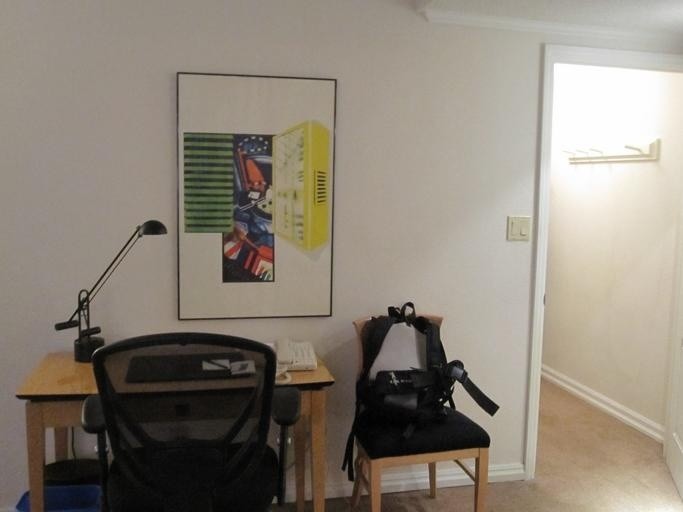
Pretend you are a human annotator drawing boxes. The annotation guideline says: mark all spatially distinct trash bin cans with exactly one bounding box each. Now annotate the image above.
[15,458,108,512]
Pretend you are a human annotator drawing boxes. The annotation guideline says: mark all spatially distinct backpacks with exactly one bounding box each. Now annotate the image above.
[354,302,447,435]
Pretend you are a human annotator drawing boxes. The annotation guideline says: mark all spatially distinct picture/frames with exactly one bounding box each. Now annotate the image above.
[177,73,338,321]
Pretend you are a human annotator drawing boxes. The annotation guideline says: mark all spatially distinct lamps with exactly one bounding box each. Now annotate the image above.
[54,213,170,364]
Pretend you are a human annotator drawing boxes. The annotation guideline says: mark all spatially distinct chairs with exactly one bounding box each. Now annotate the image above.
[80,331,300,511]
[349,309,492,510]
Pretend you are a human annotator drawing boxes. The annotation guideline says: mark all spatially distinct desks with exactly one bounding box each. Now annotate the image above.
[16,351,337,512]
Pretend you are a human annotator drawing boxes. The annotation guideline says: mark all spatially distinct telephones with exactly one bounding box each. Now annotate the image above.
[264,339,318,371]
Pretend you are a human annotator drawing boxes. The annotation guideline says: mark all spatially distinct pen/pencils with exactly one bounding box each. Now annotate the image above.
[203,359,228,370]
